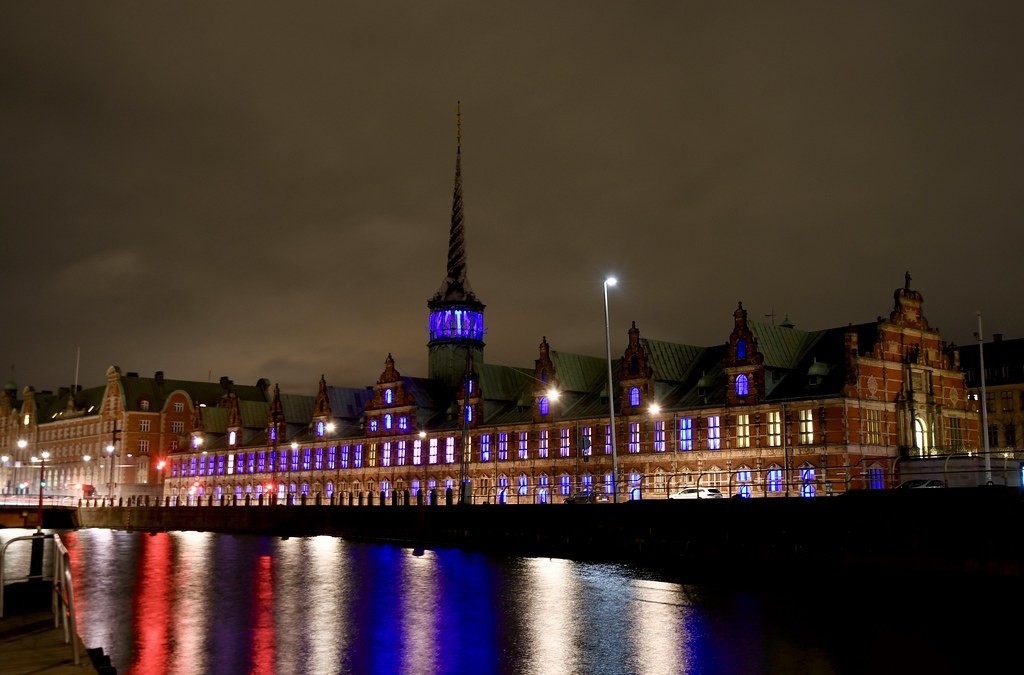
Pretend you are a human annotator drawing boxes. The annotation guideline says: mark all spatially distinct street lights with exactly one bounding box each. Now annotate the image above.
[603,276,618,502]
[976,310,993,486]
